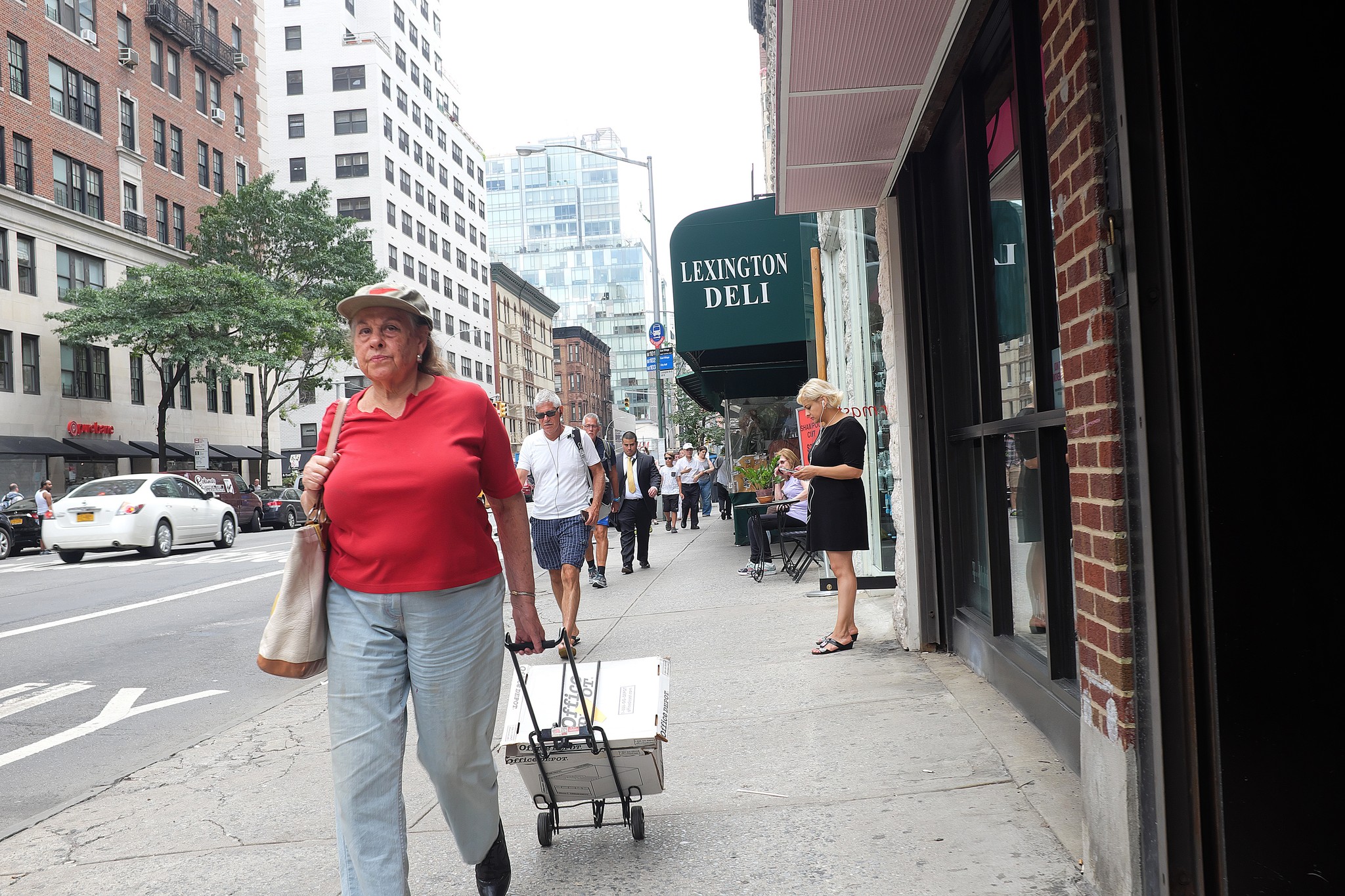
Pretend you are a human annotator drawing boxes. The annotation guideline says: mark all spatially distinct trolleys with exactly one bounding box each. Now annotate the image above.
[501,628,646,846]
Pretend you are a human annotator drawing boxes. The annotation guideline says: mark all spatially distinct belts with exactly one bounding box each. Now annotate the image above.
[682,483,698,487]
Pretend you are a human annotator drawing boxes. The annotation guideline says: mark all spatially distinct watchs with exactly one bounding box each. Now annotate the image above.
[613,497,621,502]
[697,474,701,477]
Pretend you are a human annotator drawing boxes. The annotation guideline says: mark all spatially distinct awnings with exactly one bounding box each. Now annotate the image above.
[675,195,820,417]
[988,196,1034,347]
[0,437,289,460]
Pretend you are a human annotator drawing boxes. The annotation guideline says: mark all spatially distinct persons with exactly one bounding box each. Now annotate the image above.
[299,280,539,896]
[579,412,621,588]
[615,431,662,574]
[640,446,661,525]
[34,479,54,555]
[675,443,704,529]
[1013,379,1047,635]
[713,446,733,520]
[736,447,810,577]
[695,446,715,517]
[514,388,606,659]
[789,377,868,655]
[252,479,262,493]
[1,483,25,510]
[659,452,685,533]
[678,448,686,458]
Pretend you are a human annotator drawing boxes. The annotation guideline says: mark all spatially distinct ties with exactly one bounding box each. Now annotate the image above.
[627,456,636,494]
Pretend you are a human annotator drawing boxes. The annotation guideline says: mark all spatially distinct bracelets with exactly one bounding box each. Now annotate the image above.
[509,590,536,598]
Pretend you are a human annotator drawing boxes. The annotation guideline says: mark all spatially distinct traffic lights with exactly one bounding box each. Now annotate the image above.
[499,402,507,417]
[624,397,630,411]
[492,403,497,413]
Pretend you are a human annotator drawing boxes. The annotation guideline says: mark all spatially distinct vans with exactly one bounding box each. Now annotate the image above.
[157,470,264,533]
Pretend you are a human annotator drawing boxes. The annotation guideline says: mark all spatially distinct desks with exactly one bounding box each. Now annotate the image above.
[734,499,800,583]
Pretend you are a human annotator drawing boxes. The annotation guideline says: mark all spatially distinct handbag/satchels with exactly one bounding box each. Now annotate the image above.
[607,452,642,528]
[257,397,351,678]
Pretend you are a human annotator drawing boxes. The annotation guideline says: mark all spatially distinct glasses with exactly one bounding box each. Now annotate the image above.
[640,449,647,452]
[664,457,673,460]
[535,406,560,419]
[777,459,788,464]
[582,424,598,428]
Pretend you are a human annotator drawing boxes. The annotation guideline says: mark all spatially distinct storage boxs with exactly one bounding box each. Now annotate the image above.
[732,454,767,475]
[733,472,767,492]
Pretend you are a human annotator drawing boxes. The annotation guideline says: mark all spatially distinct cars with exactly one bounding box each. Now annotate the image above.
[0,493,70,557]
[42,474,238,564]
[0,512,16,560]
[253,488,317,530]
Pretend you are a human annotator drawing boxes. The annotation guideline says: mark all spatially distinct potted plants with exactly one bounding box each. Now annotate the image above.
[733,455,787,505]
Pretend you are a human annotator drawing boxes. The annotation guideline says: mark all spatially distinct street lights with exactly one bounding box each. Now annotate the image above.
[605,416,628,441]
[515,143,667,438]
[439,329,481,360]
[635,423,657,434]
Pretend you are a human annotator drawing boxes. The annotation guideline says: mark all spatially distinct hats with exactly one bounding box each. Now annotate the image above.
[683,443,694,450]
[336,280,433,331]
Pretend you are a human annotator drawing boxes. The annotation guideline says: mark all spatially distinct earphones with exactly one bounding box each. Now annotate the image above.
[822,400,825,407]
[560,412,561,416]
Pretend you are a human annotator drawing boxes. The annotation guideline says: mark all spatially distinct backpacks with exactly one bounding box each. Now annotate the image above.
[2,494,24,511]
[585,481,613,522]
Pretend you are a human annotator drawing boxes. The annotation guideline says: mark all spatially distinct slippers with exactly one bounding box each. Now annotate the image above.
[571,636,580,646]
[812,638,854,655]
[816,631,859,645]
[558,642,576,658]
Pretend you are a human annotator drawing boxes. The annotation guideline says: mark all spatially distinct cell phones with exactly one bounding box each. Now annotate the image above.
[580,510,589,521]
[780,468,797,474]
[778,469,784,475]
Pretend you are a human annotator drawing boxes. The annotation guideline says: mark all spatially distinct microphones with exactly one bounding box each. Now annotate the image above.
[559,436,560,440]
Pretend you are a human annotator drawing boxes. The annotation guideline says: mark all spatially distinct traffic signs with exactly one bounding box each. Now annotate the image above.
[646,347,674,372]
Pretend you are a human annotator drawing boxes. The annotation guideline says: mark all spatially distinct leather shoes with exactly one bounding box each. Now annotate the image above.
[475,815,512,896]
[654,523,658,525]
[622,565,633,574]
[681,521,687,528]
[727,516,732,519]
[721,510,726,520]
[691,526,700,529]
[639,561,650,568]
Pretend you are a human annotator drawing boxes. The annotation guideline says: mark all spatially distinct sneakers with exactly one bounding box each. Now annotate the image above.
[592,574,607,588]
[634,530,637,537]
[737,559,761,576]
[666,517,672,531]
[747,560,777,577]
[588,564,597,584]
[39,549,57,555]
[671,527,677,533]
[649,526,652,532]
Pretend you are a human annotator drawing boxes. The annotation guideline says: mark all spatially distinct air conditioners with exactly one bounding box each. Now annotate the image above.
[234,53,249,67]
[235,125,244,136]
[82,29,97,44]
[211,108,225,122]
[118,48,139,65]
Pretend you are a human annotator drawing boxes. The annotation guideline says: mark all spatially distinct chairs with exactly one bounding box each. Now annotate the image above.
[780,527,828,565]
[780,530,824,584]
